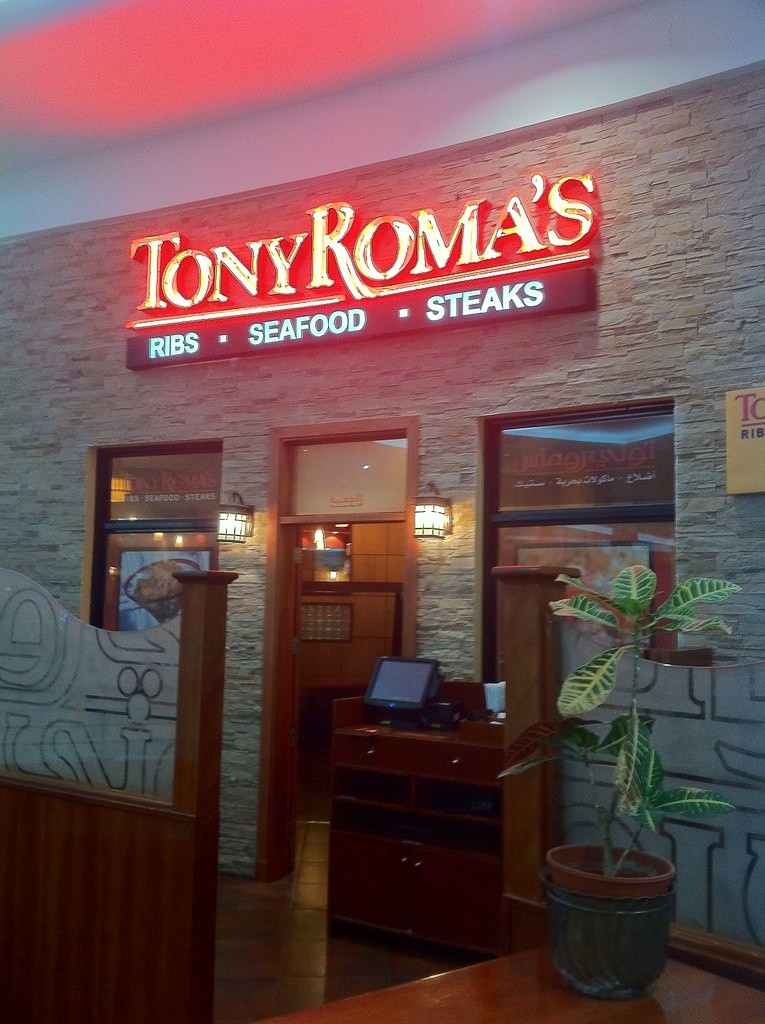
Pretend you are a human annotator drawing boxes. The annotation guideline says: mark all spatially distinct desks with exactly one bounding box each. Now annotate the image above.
[251,943,765,1024]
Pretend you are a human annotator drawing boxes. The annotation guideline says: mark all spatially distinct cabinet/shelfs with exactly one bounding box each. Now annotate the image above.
[325,683,502,964]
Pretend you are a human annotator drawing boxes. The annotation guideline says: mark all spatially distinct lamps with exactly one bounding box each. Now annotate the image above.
[216,493,253,544]
[414,481,453,538]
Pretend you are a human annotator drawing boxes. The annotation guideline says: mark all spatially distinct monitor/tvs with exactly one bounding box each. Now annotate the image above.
[364,655,437,709]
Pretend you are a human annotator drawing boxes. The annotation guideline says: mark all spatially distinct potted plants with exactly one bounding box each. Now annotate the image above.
[495,565,743,1000]
